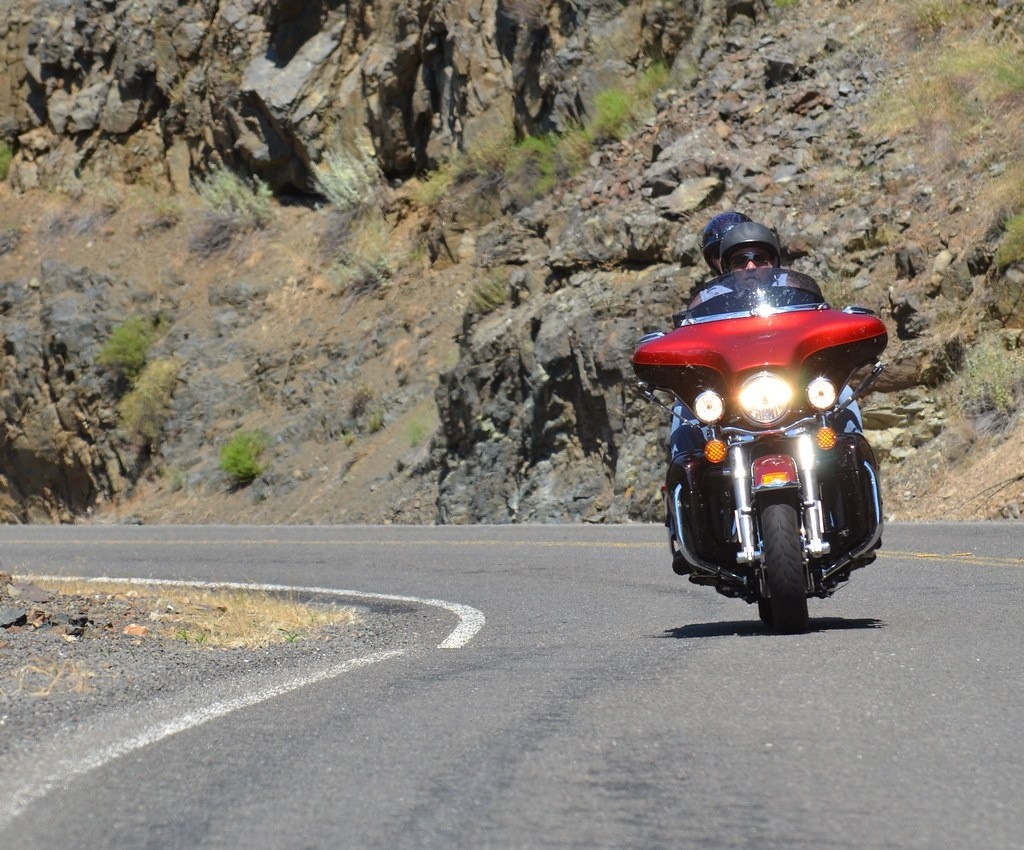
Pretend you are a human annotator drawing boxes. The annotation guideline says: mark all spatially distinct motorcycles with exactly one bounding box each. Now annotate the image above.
[628,267,889,635]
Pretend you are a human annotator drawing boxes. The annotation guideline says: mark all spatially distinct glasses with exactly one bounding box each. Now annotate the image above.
[732,251,771,266]
[712,249,720,259]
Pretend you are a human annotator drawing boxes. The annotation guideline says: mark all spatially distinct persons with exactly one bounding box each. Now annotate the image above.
[668,212,878,576]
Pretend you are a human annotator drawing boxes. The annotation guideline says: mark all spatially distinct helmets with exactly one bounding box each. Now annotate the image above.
[702,212,752,268]
[721,221,782,272]
